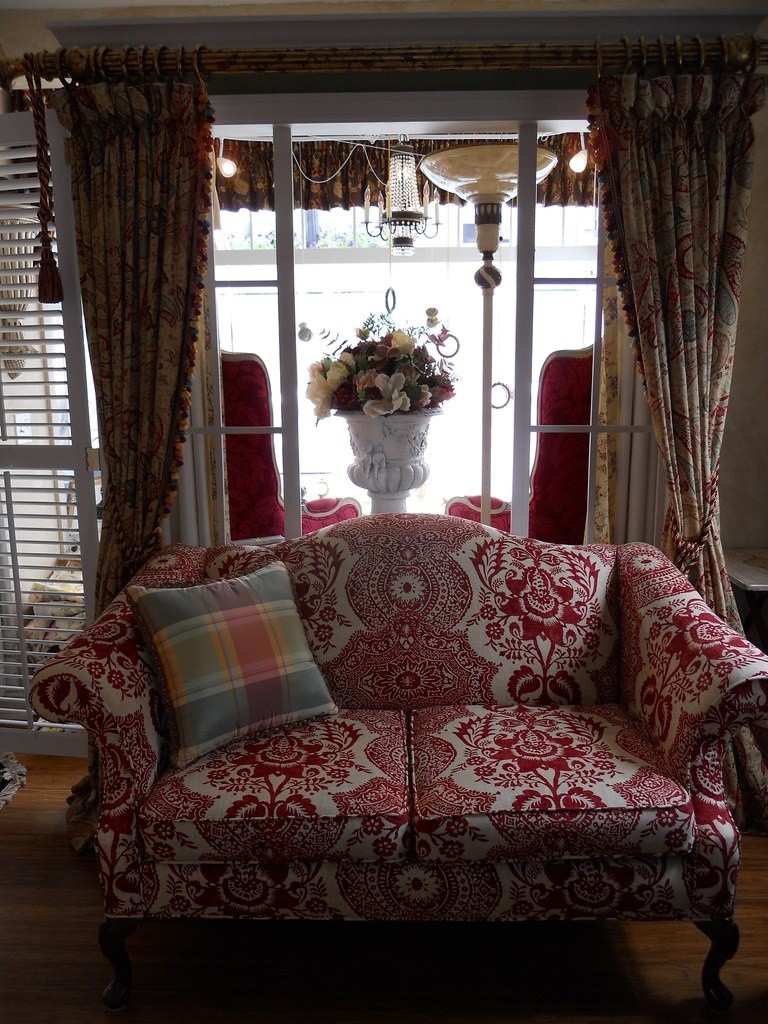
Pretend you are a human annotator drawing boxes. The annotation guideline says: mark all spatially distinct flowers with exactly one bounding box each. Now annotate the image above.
[305,306,460,427]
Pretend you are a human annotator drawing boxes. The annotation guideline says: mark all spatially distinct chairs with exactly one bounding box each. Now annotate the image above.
[26,514,768,1010]
[444,342,594,546]
[219,349,362,541]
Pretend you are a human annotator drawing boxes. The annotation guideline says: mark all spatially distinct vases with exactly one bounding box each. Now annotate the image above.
[332,405,443,518]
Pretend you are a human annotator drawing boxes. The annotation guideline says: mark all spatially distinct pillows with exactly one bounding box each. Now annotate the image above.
[127,560,338,770]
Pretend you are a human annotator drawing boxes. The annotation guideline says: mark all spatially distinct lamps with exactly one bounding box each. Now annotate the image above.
[417,144,558,523]
[360,134,443,256]
